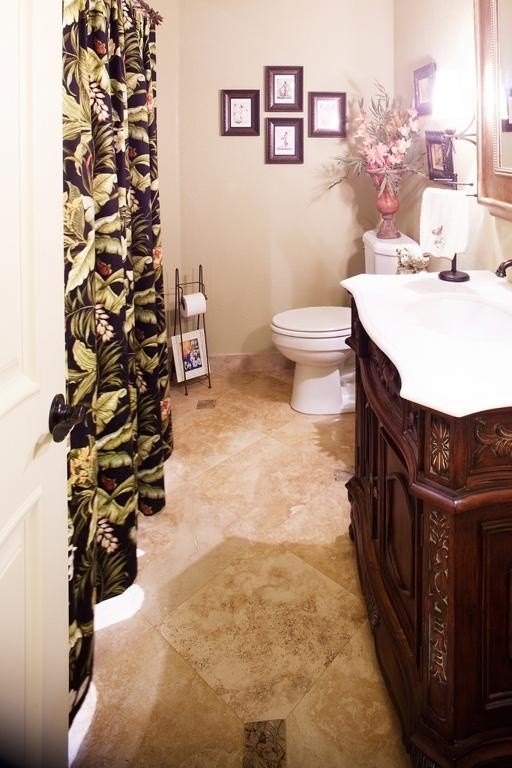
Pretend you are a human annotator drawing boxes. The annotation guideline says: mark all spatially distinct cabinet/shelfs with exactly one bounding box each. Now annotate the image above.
[341,290,510,768]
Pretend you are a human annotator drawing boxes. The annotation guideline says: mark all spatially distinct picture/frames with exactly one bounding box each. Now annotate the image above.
[223,64,348,164]
[171,328,212,384]
[411,64,455,188]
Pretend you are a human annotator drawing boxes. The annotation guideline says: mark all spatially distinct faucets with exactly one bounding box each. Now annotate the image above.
[494,258,512,278]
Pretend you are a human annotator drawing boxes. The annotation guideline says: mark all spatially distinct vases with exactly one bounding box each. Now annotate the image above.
[362,168,406,241]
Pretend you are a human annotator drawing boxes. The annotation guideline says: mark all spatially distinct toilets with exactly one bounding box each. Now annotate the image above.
[270,228,425,416]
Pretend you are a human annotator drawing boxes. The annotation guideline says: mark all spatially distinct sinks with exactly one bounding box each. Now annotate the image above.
[340,269,512,419]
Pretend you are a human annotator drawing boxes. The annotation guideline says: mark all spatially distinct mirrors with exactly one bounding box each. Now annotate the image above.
[474,0,512,217]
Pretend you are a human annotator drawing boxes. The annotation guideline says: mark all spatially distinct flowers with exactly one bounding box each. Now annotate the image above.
[325,74,478,202]
